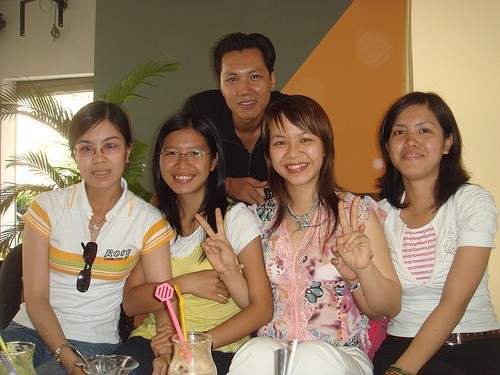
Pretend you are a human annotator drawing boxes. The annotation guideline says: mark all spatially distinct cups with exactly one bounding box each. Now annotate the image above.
[77,353,139,375]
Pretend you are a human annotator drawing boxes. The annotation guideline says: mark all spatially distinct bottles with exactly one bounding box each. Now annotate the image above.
[0,341,37,375]
[167,332,218,375]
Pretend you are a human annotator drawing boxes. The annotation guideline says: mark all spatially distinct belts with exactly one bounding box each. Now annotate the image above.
[388,329,500,345]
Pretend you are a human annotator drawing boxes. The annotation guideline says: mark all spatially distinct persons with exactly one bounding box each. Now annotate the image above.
[2,33,500,375]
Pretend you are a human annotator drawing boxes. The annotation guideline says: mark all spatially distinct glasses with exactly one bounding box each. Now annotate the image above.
[72,142,130,157]
[159,149,213,161]
[77,241,97,292]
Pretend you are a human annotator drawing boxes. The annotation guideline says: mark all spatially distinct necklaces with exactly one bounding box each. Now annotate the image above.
[285,196,321,232]
[89,217,104,233]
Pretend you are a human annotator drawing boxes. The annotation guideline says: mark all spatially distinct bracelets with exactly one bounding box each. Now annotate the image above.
[349,282,360,293]
[385,365,414,375]
[54,343,76,366]
